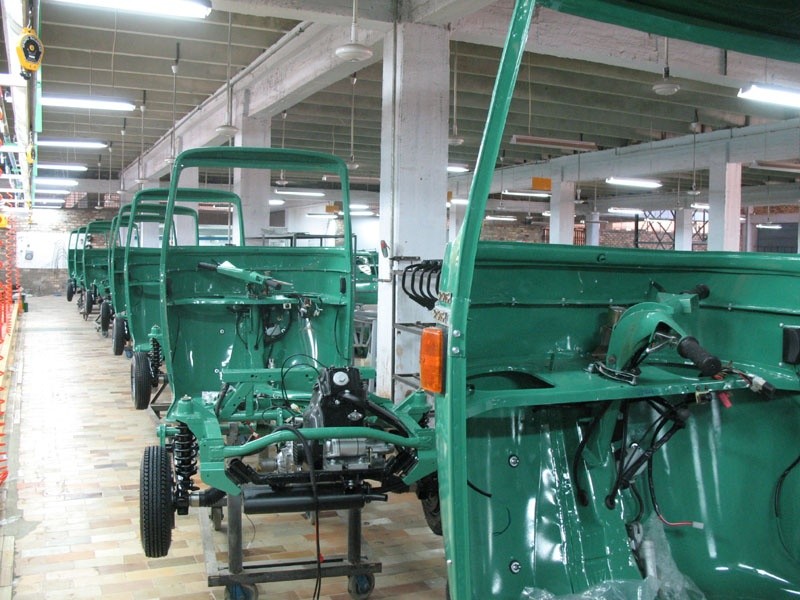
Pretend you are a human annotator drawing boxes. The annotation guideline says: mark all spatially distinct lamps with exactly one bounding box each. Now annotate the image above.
[321,127,380,186]
[756,223,783,230]
[37,52,108,150]
[737,58,800,108]
[605,176,663,188]
[42,0,213,19]
[274,139,328,197]
[484,212,518,221]
[38,111,89,172]
[509,53,598,152]
[306,212,338,218]
[502,163,552,197]
[690,173,709,210]
[32,148,78,210]
[607,188,644,214]
[4,13,137,113]
